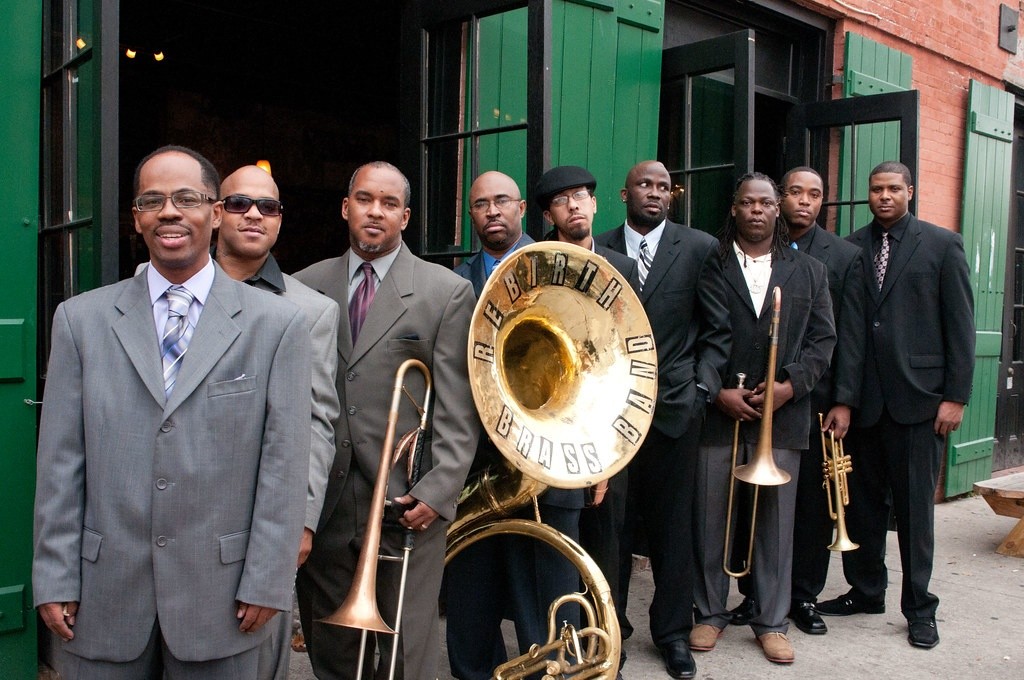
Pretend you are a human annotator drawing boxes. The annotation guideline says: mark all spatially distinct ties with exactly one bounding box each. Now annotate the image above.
[638,238,654,292]
[873,232,890,292]
[162,288,195,400]
[348,263,375,349]
[790,242,799,250]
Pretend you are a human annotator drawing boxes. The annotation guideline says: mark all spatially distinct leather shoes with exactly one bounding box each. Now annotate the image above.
[663,639,697,678]
[787,600,827,634]
[729,597,755,626]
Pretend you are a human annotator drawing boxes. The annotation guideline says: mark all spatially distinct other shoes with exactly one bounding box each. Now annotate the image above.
[688,624,722,650]
[755,632,795,663]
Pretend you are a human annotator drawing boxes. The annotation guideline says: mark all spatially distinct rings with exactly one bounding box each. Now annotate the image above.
[422,524,427,529]
[757,407,759,412]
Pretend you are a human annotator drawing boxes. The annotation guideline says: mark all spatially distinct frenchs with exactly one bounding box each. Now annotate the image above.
[441,238,662,680]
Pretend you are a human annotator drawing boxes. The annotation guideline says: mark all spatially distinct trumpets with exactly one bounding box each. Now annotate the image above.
[818,411,861,553]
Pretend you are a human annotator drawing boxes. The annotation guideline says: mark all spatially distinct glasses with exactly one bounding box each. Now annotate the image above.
[220,195,284,216]
[471,198,521,212]
[552,190,590,207]
[132,191,217,212]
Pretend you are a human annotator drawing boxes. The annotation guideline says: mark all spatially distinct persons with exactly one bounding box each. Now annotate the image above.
[531,165,643,670]
[596,160,732,680]
[291,161,482,680]
[31,145,312,680]
[446,171,585,680]
[690,173,837,663]
[135,165,340,680]
[815,161,975,648]
[730,167,866,634]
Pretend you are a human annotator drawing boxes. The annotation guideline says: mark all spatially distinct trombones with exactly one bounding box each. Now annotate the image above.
[311,356,433,680]
[722,284,793,579]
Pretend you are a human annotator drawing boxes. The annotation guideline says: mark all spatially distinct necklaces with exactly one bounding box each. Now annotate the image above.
[738,244,772,294]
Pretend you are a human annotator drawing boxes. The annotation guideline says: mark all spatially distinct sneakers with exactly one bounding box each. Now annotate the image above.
[815,593,885,616]
[908,617,940,647]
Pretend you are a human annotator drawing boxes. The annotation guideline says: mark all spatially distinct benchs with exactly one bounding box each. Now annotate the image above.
[973,473,1024,558]
